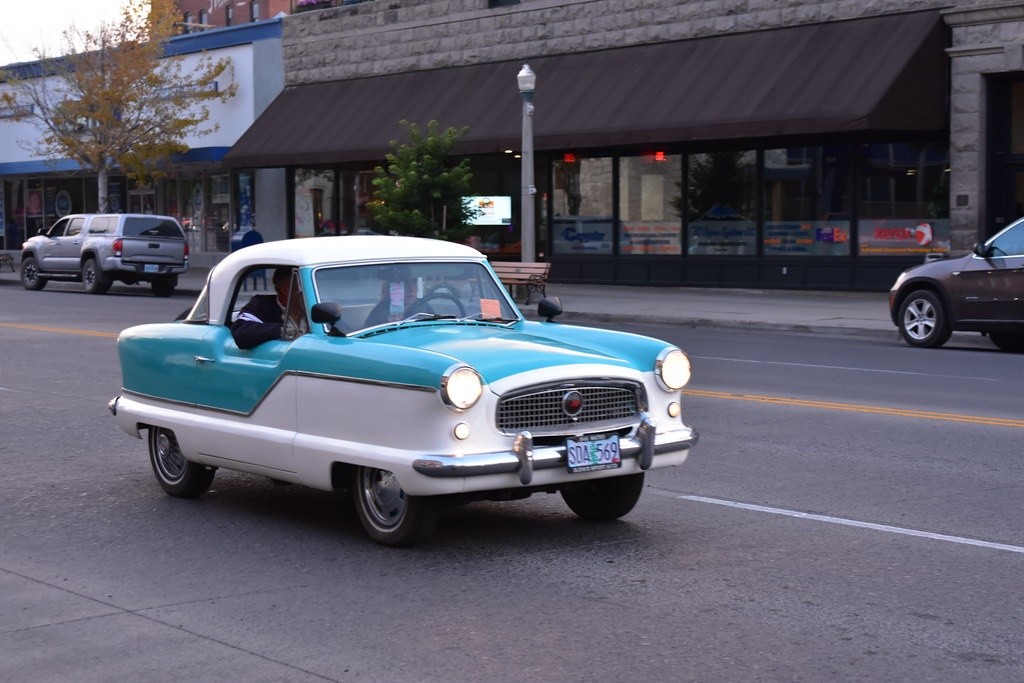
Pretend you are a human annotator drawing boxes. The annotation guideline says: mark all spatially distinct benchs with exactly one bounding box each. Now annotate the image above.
[467,261,551,306]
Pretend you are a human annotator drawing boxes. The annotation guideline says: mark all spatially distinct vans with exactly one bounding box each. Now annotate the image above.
[553,215,634,258]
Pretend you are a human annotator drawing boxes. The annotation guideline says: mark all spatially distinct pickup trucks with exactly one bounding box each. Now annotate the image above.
[20,213,190,297]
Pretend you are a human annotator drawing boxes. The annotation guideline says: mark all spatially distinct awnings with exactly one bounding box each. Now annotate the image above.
[221,6,955,170]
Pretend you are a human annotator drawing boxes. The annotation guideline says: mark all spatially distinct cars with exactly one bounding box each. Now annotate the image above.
[109,235,700,549]
[888,216,1024,351]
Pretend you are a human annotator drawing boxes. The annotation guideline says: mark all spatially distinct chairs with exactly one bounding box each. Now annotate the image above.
[340,304,378,331]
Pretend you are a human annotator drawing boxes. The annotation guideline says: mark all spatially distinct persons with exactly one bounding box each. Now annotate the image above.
[230,267,352,350]
[364,277,434,331]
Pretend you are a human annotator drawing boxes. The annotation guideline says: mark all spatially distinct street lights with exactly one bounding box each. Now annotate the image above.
[515,64,545,302]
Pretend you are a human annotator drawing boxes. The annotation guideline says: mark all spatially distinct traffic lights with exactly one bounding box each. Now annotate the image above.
[651,151,667,166]
[559,152,579,167]
